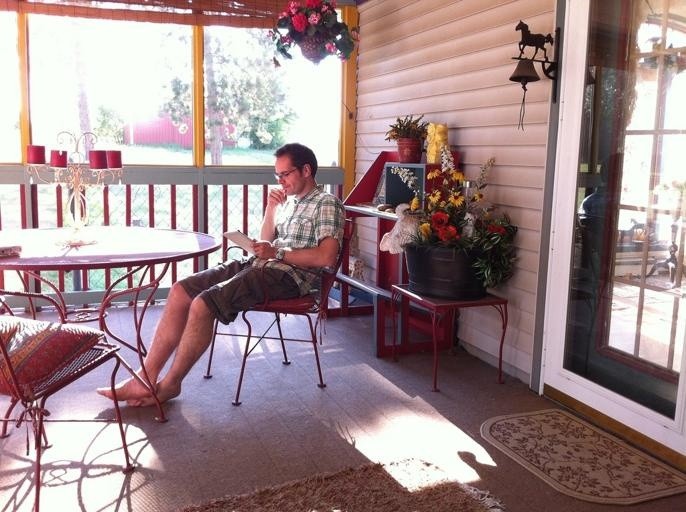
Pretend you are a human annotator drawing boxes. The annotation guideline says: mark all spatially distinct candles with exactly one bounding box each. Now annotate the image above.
[26,144,123,170]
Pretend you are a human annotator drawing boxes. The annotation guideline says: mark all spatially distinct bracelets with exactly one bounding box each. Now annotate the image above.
[275,248,285,261]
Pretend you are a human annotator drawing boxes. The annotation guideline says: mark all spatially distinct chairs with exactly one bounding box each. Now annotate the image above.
[204,217,356,406]
[0,310,135,511]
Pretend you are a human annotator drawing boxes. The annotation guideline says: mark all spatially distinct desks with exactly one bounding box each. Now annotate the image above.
[390,284,509,394]
[0,223,223,423]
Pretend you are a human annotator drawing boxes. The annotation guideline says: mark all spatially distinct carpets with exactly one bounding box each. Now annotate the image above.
[479,407,686,506]
[168,455,506,512]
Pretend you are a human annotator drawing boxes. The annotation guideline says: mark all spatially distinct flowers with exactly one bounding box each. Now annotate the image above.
[387,143,521,291]
[266,0,356,70]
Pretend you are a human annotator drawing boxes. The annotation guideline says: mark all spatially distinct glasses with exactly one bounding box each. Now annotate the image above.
[273,168,298,180]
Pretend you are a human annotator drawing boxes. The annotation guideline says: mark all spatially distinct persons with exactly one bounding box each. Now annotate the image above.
[94,141,348,409]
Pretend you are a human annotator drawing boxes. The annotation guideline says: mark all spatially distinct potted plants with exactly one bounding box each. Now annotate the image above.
[384,112,431,163]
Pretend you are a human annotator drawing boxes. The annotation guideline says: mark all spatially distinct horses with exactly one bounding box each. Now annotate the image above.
[515,18,553,61]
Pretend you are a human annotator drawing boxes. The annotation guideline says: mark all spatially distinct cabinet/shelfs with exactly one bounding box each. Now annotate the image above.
[331,202,401,310]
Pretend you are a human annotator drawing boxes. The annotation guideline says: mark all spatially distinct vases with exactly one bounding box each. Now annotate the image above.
[296,34,332,65]
[398,238,487,300]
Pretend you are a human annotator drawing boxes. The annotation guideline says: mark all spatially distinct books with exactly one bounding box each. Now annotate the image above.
[222,229,256,255]
